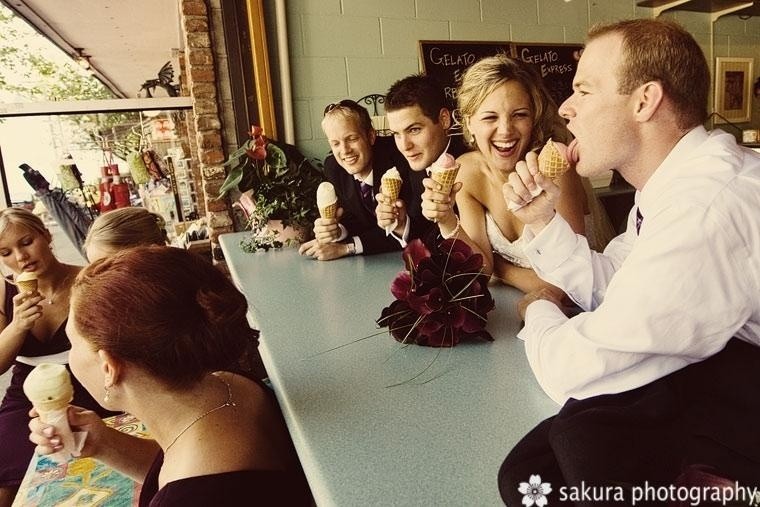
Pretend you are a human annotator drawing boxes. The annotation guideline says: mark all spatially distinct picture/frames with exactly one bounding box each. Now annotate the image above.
[715,56,754,122]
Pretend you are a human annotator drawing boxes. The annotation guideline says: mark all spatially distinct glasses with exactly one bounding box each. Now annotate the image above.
[323,99,358,116]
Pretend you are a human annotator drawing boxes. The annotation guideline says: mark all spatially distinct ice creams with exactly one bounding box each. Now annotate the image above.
[506,138,576,212]
[16,271,38,303]
[425,137,462,224]
[381,166,403,237]
[316,182,338,218]
[22,362,74,460]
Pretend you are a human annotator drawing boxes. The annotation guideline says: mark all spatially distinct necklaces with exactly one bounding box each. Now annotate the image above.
[39,265,70,305]
[157,370,237,457]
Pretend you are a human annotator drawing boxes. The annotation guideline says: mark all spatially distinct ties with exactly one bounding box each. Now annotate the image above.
[360,181,377,219]
[636,207,644,238]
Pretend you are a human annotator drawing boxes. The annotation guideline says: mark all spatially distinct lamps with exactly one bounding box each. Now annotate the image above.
[138,60,186,119]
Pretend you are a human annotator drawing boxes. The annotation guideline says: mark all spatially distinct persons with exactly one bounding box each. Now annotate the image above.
[296,95,411,263]
[497,16,758,411]
[27,242,317,506]
[0,205,91,507]
[372,69,472,252]
[418,51,621,305]
[79,206,172,265]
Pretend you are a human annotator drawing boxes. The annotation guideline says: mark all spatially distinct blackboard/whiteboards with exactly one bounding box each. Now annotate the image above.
[417,39,586,161]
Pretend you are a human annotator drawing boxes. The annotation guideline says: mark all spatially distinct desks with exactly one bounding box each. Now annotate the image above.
[216,229,563,506]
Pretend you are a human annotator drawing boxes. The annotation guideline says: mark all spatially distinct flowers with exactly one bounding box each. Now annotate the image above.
[213,124,326,254]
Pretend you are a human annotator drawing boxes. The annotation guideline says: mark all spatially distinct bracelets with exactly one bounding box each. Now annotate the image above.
[438,214,461,240]
